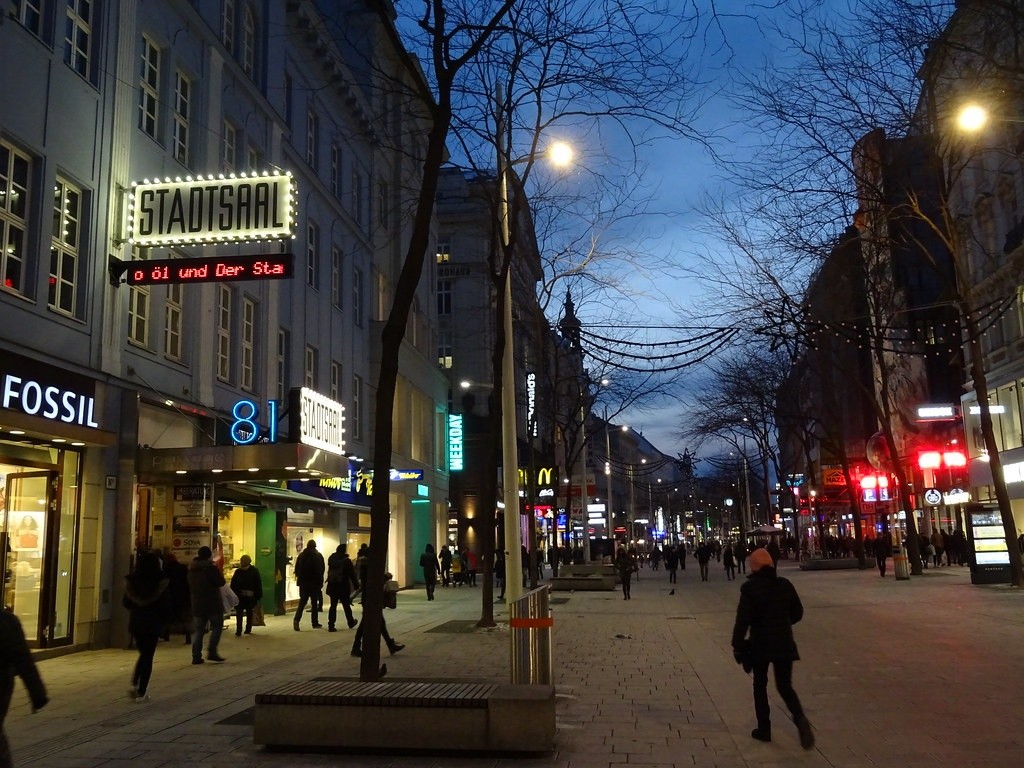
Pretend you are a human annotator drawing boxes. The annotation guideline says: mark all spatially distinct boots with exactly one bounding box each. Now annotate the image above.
[387,638,405,655]
[350,641,362,656]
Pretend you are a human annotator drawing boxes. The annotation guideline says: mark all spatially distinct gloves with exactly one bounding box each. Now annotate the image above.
[733,648,742,664]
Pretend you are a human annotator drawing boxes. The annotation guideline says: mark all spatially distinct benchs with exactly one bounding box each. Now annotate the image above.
[810,557,875,569]
[550,577,615,590]
[254,679,556,753]
[559,564,616,576]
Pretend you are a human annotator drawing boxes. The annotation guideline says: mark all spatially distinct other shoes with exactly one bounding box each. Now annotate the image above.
[136,694,150,702]
[329,627,336,631]
[193,659,204,664]
[497,596,503,600]
[313,624,322,628]
[752,728,771,741]
[294,619,300,631]
[796,716,816,751]
[427,590,434,600]
[349,619,357,628]
[208,654,226,662]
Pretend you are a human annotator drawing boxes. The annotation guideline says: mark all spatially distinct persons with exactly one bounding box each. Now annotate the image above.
[420,543,478,601]
[547,547,584,569]
[1018,534,1024,563]
[731,548,815,751]
[123,553,171,703]
[0,612,50,768]
[825,534,856,558]
[614,548,644,600]
[864,532,889,577]
[789,534,808,553]
[325,543,405,656]
[495,545,544,599]
[652,543,686,583]
[230,556,263,636]
[919,528,970,569]
[160,546,226,664]
[294,540,325,631]
[694,540,748,581]
[755,540,780,568]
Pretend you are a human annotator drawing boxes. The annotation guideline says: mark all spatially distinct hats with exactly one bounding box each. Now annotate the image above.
[198,546,211,559]
[749,549,773,570]
[241,555,251,565]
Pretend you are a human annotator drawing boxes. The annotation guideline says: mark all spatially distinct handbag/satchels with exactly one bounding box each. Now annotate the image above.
[220,584,239,610]
[384,579,398,609]
[252,604,265,626]
[928,544,936,556]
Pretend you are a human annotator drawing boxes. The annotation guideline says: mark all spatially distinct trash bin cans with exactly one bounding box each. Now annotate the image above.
[508,585,552,684]
